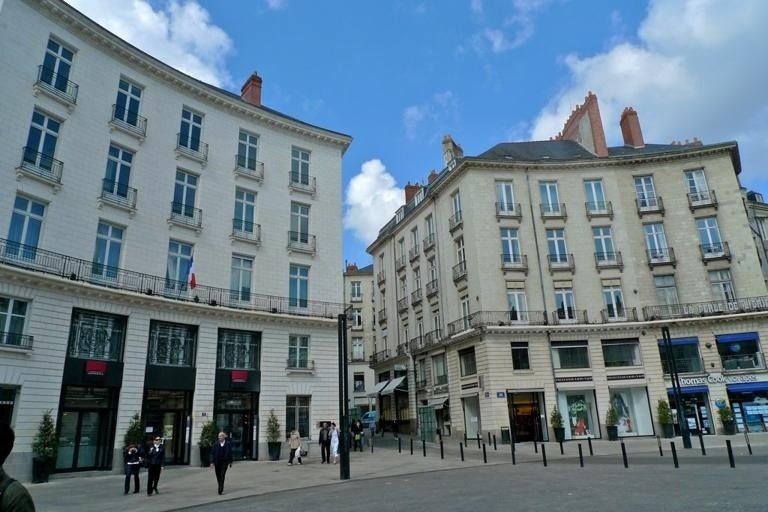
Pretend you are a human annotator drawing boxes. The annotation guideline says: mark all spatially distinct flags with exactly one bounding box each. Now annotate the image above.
[186,255,198,292]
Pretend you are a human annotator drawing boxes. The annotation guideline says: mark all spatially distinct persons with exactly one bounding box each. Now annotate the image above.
[125,437,140,494]
[0,424,36,512]
[353,420,364,452]
[147,435,165,496]
[212,431,233,494]
[288,427,303,465]
[318,422,342,465]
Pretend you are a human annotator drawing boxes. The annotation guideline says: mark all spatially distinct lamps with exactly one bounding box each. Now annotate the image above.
[85,360,108,377]
[231,370,250,384]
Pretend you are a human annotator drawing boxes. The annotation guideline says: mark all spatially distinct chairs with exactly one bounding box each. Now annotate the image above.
[739,357,755,369]
[724,359,736,369]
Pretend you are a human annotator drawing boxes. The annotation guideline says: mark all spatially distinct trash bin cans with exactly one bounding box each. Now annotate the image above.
[501,426,510,444]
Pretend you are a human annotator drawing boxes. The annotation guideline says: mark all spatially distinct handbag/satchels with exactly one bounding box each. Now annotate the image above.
[295,446,307,458]
[354,434,361,441]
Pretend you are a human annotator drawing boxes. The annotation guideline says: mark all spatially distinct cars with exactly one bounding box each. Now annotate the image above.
[359,410,376,428]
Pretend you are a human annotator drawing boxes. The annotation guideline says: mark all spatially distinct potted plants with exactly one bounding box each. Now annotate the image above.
[31,408,57,483]
[717,398,737,435]
[264,408,281,461]
[657,397,674,438]
[551,404,565,442]
[604,404,619,441]
[123,411,146,474]
[197,421,219,467]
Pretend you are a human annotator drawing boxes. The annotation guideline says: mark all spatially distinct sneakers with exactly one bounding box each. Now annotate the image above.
[288,463,301,466]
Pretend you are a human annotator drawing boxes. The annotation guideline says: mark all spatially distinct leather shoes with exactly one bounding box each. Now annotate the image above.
[123,485,159,496]
[320,460,338,465]
